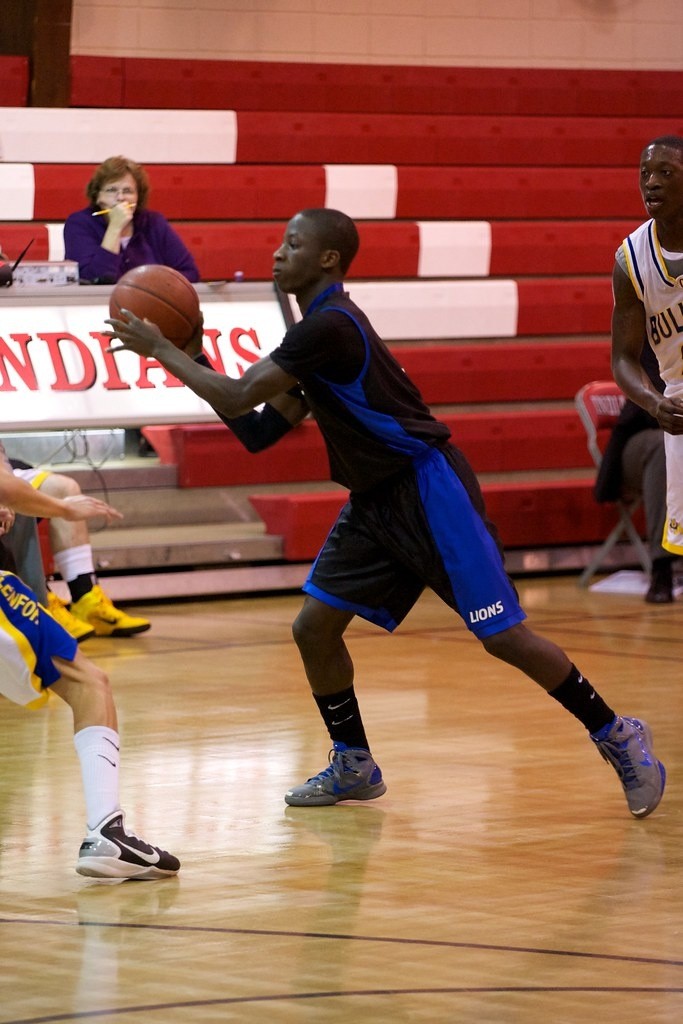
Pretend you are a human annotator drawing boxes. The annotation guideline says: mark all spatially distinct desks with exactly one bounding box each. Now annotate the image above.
[0,280,303,432]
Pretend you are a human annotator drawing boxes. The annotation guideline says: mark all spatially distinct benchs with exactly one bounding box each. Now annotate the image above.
[0,53,683,598]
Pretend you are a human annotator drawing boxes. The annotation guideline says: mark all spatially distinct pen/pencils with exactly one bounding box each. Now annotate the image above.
[91,203,136,216]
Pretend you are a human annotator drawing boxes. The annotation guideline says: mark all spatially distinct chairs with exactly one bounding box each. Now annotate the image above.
[576,381,652,588]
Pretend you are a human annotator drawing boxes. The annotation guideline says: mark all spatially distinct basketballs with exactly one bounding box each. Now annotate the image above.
[110,263,200,354]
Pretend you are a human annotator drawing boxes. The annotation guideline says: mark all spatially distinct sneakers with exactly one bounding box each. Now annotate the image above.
[46,583,150,643]
[285,742,388,806]
[590,716,666,817]
[75,809,180,880]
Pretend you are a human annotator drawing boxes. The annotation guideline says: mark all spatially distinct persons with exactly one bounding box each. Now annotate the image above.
[99,207,667,819]
[64,158,203,284]
[1,435,182,880]
[591,336,683,603]
[614,136,683,556]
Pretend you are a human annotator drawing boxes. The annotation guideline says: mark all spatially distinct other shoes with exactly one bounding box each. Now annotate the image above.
[645,557,673,603]
